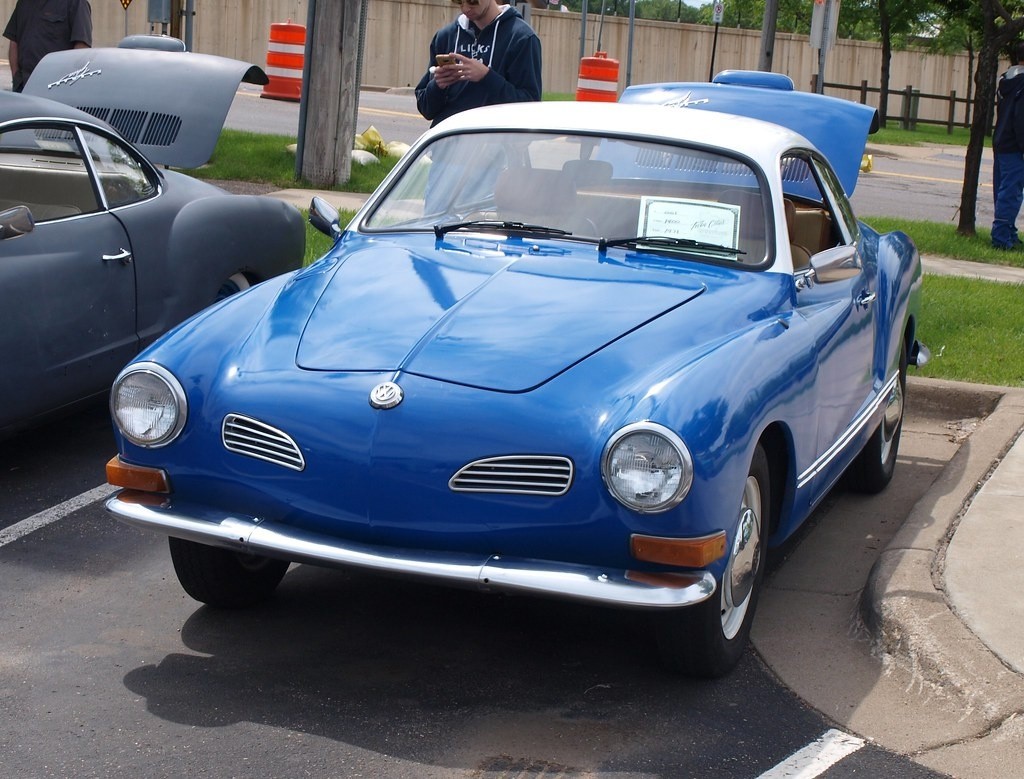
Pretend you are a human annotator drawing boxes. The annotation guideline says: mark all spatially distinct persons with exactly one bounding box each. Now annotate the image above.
[415,0,543,217]
[990,37,1024,251]
[2,0,94,93]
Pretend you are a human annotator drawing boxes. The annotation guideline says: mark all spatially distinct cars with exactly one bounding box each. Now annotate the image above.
[104,70,933,678]
[0,35,307,439]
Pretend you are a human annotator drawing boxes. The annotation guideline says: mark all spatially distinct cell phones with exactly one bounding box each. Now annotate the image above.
[436,54,456,67]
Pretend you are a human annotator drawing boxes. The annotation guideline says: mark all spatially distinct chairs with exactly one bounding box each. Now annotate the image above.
[719,188,813,274]
[462,167,598,239]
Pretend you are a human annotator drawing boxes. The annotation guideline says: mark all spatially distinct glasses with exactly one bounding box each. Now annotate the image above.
[452,0,482,5]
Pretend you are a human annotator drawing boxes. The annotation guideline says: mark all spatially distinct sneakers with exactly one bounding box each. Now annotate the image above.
[993,239,1023,252]
